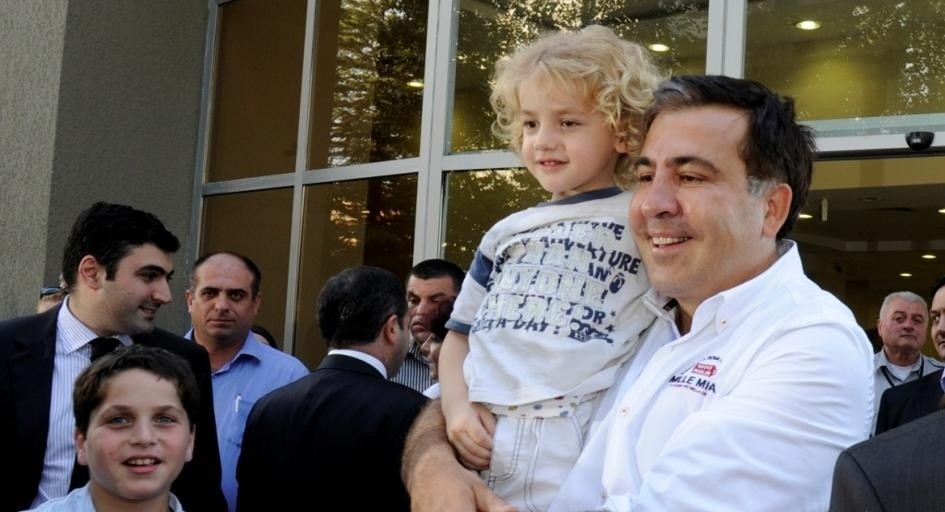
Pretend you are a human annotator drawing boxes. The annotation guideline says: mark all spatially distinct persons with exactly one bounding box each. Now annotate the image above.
[175,252,308,509]
[238,266,435,510]
[438,26,659,511]
[390,260,464,395]
[24,341,202,512]
[402,74,871,511]
[419,300,454,398]
[37,285,69,313]
[827,403,945,512]
[248,324,279,351]
[0,199,230,512]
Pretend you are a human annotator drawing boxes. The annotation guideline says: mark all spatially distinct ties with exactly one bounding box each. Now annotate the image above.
[71,337,120,495]
